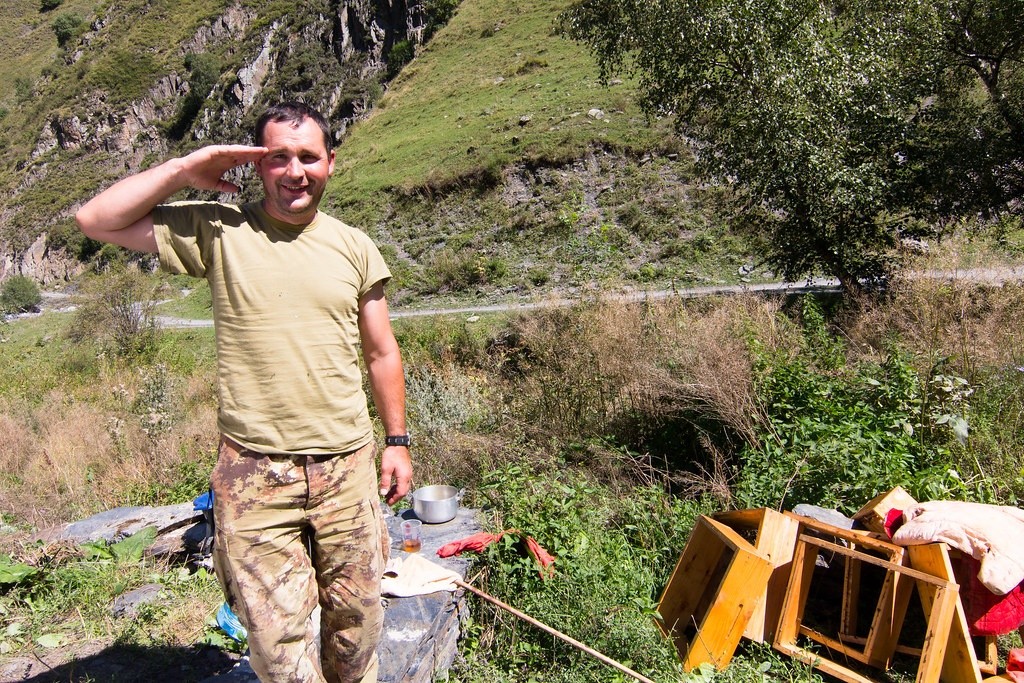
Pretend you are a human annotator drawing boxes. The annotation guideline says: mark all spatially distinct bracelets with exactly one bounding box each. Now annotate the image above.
[384,433,410,446]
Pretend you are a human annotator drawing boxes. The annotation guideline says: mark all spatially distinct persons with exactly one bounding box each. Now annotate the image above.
[77,102,412,683]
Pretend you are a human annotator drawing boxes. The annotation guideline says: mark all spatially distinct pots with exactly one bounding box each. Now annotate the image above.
[408,484,466,523]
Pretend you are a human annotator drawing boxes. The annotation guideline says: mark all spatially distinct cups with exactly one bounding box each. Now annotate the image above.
[400,519,422,553]
